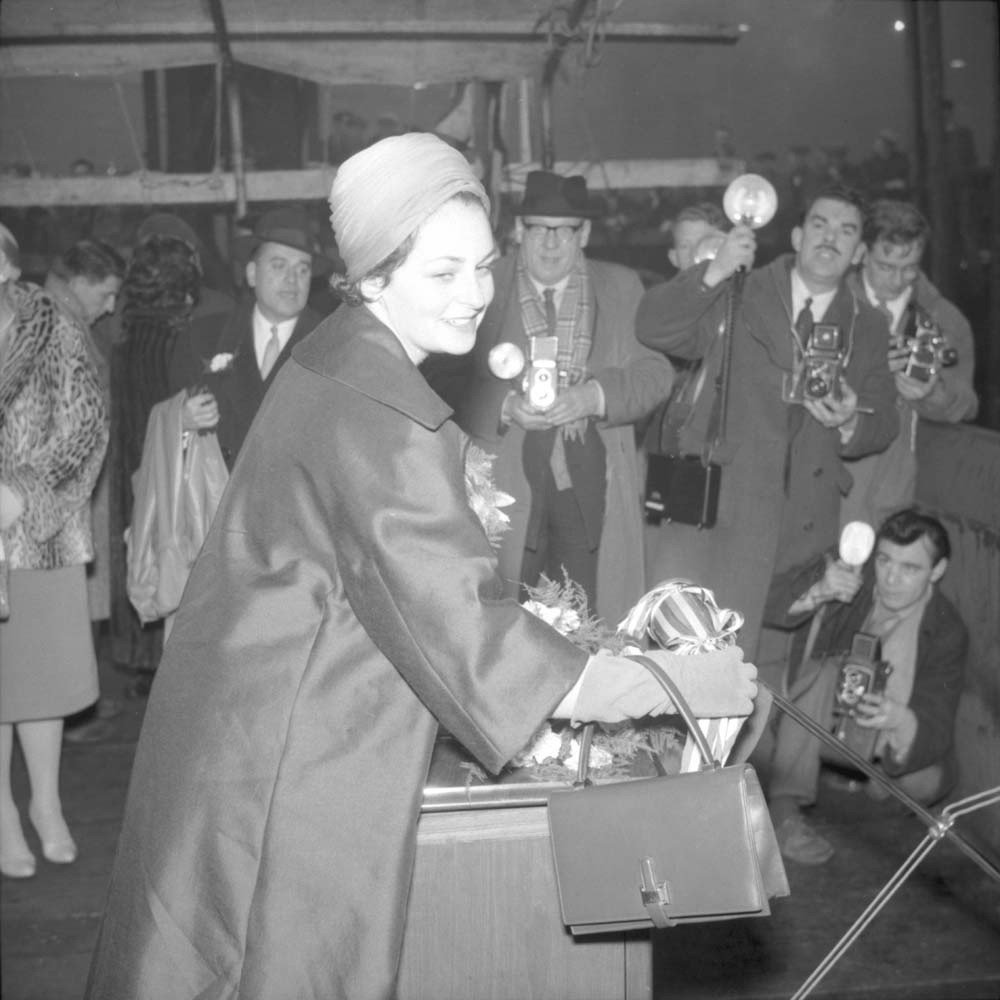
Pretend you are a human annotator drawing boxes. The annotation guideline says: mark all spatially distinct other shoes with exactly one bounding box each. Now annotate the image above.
[766,802,832,865]
[124,670,155,699]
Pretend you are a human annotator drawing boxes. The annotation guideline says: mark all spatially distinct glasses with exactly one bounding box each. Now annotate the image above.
[520,218,583,245]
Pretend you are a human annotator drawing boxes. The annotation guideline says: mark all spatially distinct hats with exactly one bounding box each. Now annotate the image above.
[132,213,206,250]
[330,130,491,280]
[248,206,319,257]
[522,171,600,217]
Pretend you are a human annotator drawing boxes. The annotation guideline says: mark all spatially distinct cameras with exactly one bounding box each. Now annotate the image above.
[802,322,846,403]
[833,632,893,718]
[893,313,958,383]
[522,335,559,413]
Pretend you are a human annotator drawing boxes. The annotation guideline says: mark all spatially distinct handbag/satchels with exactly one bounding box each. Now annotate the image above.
[642,454,717,530]
[547,654,790,936]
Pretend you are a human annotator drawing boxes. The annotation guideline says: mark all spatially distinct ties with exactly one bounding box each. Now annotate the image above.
[795,297,814,362]
[875,299,890,332]
[260,324,281,379]
[543,287,555,337]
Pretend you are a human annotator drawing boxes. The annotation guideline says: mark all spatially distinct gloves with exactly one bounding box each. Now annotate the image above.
[569,646,758,727]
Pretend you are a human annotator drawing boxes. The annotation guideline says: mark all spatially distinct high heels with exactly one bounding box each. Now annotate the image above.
[26,802,79,864]
[0,807,34,881]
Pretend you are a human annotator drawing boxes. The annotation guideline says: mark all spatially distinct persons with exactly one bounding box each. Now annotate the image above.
[85,133,759,1000]
[443,170,978,864]
[0,159,327,878]
